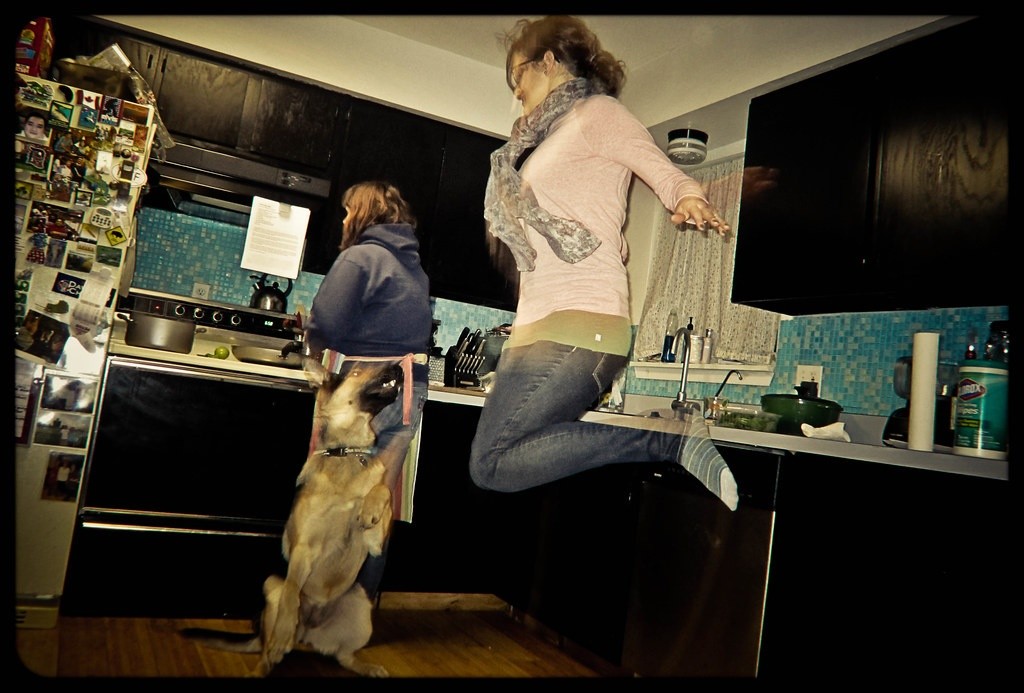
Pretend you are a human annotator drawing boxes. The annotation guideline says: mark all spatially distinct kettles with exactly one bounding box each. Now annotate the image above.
[249,273,293,314]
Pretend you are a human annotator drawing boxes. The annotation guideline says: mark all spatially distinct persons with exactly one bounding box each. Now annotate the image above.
[16,113,47,140]
[55,381,78,410]
[74,139,90,157]
[54,459,70,497]
[26,205,77,240]
[469,15,738,513]
[49,155,86,187]
[310,182,430,601]
[54,132,73,152]
[59,425,86,448]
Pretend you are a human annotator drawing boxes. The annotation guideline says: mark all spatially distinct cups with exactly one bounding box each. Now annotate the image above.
[703,396,729,420]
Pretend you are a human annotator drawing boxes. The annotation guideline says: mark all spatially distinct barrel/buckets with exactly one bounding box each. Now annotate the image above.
[953,359,1009,461]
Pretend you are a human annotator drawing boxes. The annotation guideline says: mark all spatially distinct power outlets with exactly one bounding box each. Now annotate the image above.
[795,365,823,397]
[192,283,211,300]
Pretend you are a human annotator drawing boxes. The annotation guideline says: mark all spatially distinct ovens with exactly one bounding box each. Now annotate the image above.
[60,355,318,624]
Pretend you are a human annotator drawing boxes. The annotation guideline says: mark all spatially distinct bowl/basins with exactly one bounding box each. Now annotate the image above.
[58,54,130,98]
[716,406,782,434]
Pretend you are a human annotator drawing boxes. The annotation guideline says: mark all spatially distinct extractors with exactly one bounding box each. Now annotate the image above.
[146,133,331,229]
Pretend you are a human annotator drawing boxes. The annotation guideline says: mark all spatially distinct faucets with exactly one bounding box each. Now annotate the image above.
[670,328,700,422]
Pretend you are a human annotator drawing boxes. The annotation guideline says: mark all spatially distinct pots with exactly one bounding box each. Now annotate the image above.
[117,311,208,355]
[760,393,844,438]
[232,341,306,370]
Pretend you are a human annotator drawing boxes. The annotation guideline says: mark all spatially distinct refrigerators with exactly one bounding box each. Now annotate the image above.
[12,70,158,631]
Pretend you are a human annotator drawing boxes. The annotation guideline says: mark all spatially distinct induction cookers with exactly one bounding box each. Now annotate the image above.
[108,287,309,382]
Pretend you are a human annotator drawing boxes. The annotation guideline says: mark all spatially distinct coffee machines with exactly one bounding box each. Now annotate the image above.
[881,356,959,456]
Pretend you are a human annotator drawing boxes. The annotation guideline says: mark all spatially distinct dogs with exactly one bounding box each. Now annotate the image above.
[199,345,405,678]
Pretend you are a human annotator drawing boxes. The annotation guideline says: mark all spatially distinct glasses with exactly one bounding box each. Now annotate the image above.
[508,54,561,86]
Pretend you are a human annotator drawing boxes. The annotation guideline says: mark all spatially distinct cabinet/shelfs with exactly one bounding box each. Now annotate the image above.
[45,13,518,313]
[57,356,1024,693]
[731,0,1024,317]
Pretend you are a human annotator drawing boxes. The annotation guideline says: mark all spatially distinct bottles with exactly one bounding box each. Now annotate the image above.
[661,308,678,363]
[702,328,714,364]
[689,334,703,364]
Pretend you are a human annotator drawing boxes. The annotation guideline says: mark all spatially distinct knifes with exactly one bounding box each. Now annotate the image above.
[454,326,486,377]
